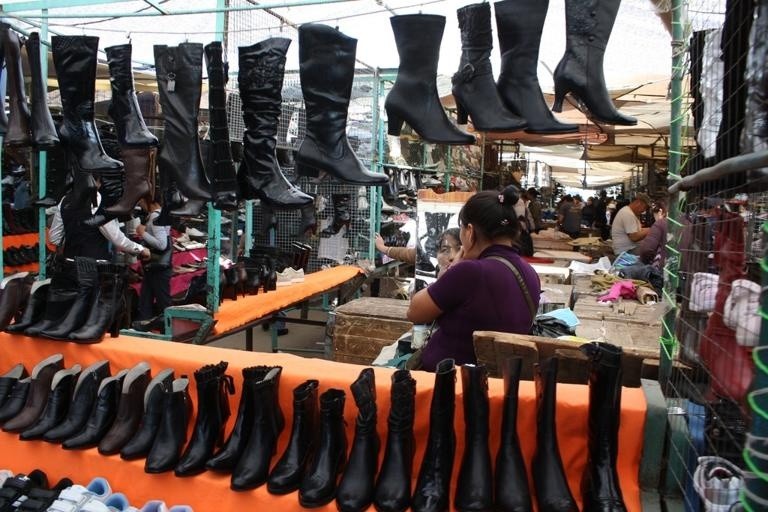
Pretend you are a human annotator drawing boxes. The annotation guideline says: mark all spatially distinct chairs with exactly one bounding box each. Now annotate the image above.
[471,330,644,388]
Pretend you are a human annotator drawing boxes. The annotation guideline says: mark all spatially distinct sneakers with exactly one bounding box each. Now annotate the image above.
[0,469,14,489]
[78,493,131,512]
[220,232,230,241]
[8,478,73,512]
[1,176,14,186]
[13,164,26,172]
[220,216,233,224]
[282,268,304,283]
[173,241,206,252]
[46,477,112,512]
[177,233,190,242]
[689,273,720,313]
[693,455,746,512]
[275,271,292,285]
[134,500,168,512]
[190,214,205,222]
[723,279,763,346]
[186,227,204,236]
[172,237,186,252]
[422,177,442,186]
[168,506,191,512]
[0,469,48,512]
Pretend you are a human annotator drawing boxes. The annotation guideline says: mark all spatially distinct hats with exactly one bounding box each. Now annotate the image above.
[636,192,653,208]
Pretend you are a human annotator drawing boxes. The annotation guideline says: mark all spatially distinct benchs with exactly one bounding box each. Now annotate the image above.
[120,265,368,345]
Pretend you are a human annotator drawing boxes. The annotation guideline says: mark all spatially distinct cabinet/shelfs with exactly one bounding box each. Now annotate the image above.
[0,331,646,512]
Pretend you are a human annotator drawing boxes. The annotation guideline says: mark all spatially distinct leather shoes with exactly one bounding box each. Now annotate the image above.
[245,268,260,295]
[2,242,50,266]
[172,260,207,277]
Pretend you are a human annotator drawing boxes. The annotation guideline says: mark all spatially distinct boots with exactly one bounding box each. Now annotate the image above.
[552,0,638,126]
[0,375,33,425]
[251,196,280,237]
[267,380,319,495]
[3,29,31,149]
[120,368,174,461]
[153,44,217,202]
[44,360,112,444]
[25,287,78,336]
[72,123,131,233]
[297,24,389,186]
[691,31,704,157]
[63,148,98,212]
[701,207,754,403]
[425,213,451,258]
[5,278,51,335]
[34,149,68,207]
[170,276,205,308]
[0,21,11,137]
[336,368,380,512]
[51,35,125,177]
[323,193,352,234]
[385,14,476,146]
[531,355,579,512]
[236,38,314,209]
[104,149,152,214]
[411,359,456,512]
[493,356,532,512]
[373,370,416,512]
[0,272,30,330]
[2,354,64,431]
[68,264,128,344]
[24,32,61,151]
[205,366,274,472]
[291,193,317,237]
[204,42,240,211]
[40,256,102,340]
[382,137,446,169]
[260,255,276,293]
[105,45,159,147]
[0,363,30,410]
[19,364,83,441]
[2,204,36,235]
[581,341,629,511]
[298,389,348,508]
[62,370,129,449]
[174,361,236,477]
[144,374,193,473]
[238,242,312,274]
[231,366,285,491]
[450,3,529,132]
[741,5,768,181]
[455,359,494,511]
[153,146,184,226]
[677,217,713,368]
[494,1,579,135]
[98,363,152,455]
[697,28,723,173]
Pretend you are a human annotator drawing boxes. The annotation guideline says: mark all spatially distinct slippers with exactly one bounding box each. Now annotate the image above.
[331,298,339,307]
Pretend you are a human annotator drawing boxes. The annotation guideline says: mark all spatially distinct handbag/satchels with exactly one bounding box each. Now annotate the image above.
[45,249,69,284]
[649,268,664,289]
[403,349,423,371]
[141,212,173,271]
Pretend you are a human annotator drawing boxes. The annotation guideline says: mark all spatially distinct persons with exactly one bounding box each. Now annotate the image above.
[374,232,416,264]
[403,183,541,372]
[527,188,541,233]
[137,193,173,319]
[436,228,462,271]
[611,192,651,257]
[556,191,629,237]
[48,174,150,261]
[640,198,691,302]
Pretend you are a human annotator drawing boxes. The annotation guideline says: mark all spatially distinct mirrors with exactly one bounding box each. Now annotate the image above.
[416,190,476,290]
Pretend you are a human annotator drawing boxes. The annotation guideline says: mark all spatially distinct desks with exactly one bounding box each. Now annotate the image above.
[526,234,683,369]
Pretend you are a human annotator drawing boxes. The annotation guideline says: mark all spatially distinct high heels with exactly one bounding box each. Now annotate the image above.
[414,170,429,188]
[223,268,240,301]
[131,314,165,335]
[200,269,227,306]
[383,167,417,210]
[228,261,249,298]
[380,229,410,247]
[341,219,366,239]
[170,186,215,217]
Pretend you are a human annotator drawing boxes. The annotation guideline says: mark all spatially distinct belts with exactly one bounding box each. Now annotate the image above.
[62,259,76,265]
